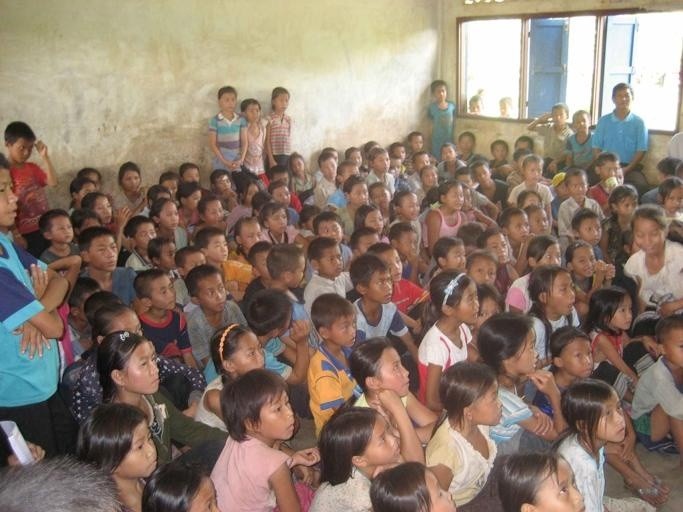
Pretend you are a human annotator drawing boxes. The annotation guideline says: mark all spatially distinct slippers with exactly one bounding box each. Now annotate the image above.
[623,474,671,507]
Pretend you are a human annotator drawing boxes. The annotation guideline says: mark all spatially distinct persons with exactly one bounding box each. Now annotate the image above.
[240,97,271,189]
[0,128,683,512]
[527,102,575,180]
[470,95,486,117]
[591,83,649,204]
[266,87,294,172]
[209,85,249,191]
[499,97,515,119]
[427,79,459,161]
[3,121,59,259]
[565,109,594,172]
